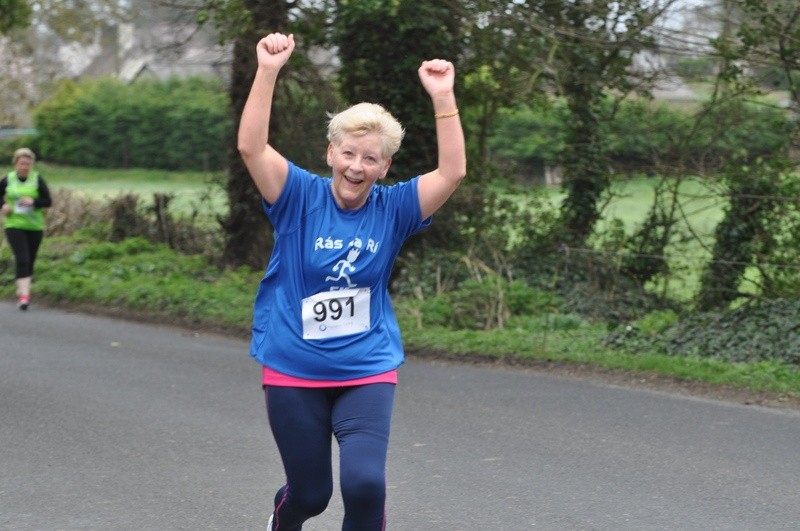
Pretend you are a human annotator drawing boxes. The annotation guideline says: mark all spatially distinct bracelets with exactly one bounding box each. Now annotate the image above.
[433,109,458,118]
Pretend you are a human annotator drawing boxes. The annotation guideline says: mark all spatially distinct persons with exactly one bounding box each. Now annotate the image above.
[237,32,466,531]
[0,147,51,311]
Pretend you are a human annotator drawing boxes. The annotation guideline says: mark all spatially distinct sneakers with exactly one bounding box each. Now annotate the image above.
[18,295,29,310]
